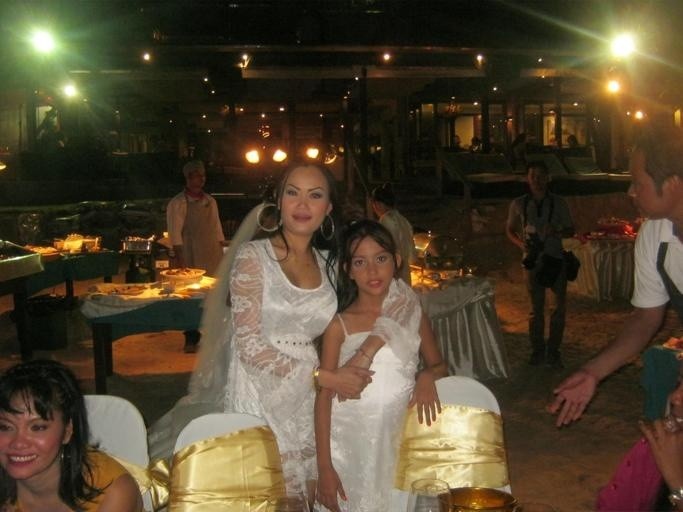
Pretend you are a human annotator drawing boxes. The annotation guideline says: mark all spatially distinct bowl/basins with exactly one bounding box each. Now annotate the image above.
[160,266,206,284]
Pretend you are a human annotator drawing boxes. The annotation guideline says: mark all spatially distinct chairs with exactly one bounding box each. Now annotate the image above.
[171,412,286,512]
[53,389,169,512]
[393,377,512,512]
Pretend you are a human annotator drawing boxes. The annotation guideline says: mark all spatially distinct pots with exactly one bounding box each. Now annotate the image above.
[435,486,519,512]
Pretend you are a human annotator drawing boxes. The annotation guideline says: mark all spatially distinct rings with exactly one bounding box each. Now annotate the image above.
[664,419,679,432]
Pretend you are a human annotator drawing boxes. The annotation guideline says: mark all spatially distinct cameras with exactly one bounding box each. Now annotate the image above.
[521,232,544,270]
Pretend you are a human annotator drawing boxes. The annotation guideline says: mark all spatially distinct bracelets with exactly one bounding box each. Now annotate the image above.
[668,487,683,506]
[313,366,321,392]
[356,347,373,364]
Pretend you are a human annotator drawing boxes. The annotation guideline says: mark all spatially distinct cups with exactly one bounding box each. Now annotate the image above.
[265,493,307,512]
[404,476,453,512]
[465,265,474,275]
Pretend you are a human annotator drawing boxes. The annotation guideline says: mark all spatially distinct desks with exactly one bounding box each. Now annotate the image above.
[408,269,510,386]
[11,247,117,307]
[0,227,639,395]
[82,277,218,395]
[560,234,633,306]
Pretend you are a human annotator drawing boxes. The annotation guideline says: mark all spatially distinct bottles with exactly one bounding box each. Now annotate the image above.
[455,263,464,277]
[425,251,431,268]
[188,142,195,157]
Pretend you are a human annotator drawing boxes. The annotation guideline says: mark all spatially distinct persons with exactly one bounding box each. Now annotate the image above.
[166,154,226,354]
[505,159,576,365]
[1,358,146,512]
[567,134,581,148]
[216,158,420,511]
[366,182,417,288]
[312,219,449,511]
[545,122,682,427]
[592,366,683,512]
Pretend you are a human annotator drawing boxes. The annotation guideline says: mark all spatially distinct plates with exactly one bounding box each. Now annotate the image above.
[104,285,143,295]
[662,338,683,352]
